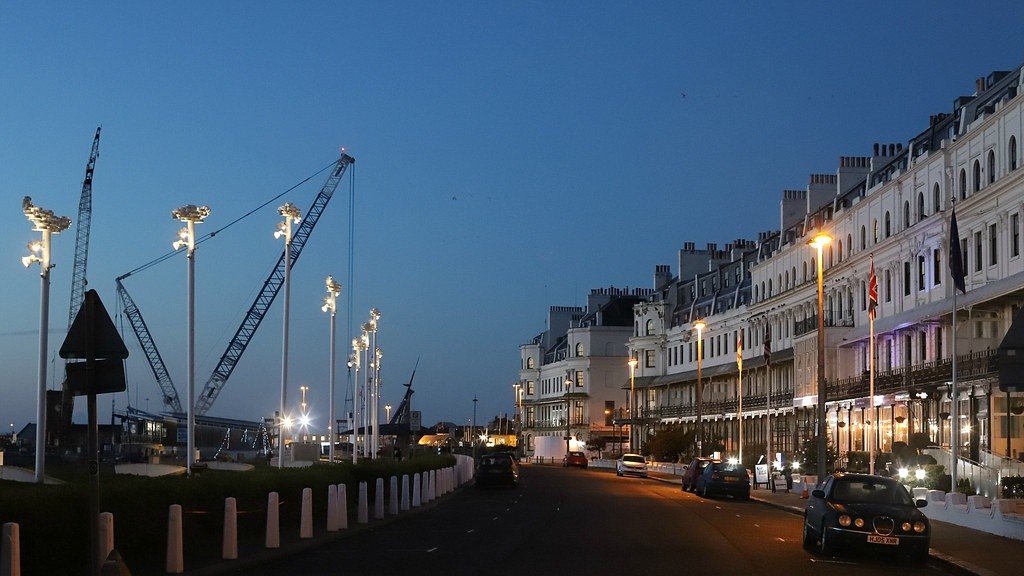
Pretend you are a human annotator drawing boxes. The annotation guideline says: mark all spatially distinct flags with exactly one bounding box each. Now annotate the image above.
[948,209,967,295]
[736,329,743,372]
[763,321,772,367]
[867,260,879,321]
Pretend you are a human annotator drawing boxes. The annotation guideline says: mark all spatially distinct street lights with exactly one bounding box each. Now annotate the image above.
[626,359,637,455]
[693,319,705,462]
[17,194,391,497]
[564,378,574,452]
[809,230,828,477]
[513,383,522,456]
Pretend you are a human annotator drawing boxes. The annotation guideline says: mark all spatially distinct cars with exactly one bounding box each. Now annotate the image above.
[804,472,929,562]
[615,453,649,479]
[562,451,588,469]
[475,453,520,487]
[489,452,519,475]
[695,463,750,502]
[681,457,723,492]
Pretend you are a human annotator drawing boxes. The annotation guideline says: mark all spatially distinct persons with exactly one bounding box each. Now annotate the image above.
[781,461,793,493]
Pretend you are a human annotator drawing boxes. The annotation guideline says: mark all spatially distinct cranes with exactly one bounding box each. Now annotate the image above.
[65,125,357,415]
